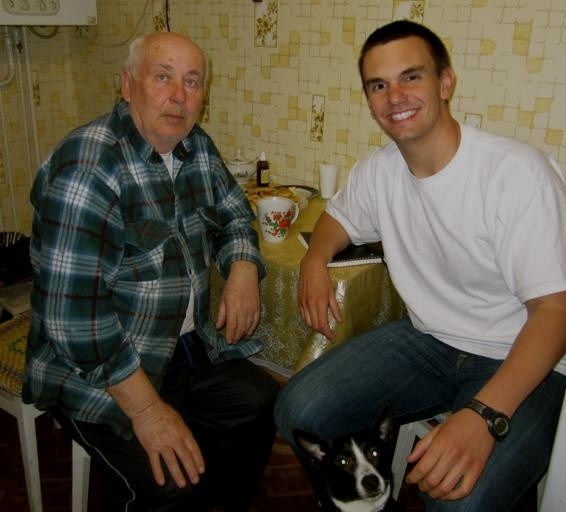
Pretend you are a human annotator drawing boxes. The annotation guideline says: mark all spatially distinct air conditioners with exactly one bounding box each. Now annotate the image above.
[292,401,405,512]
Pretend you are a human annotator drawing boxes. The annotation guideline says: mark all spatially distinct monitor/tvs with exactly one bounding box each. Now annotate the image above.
[296,230,384,268]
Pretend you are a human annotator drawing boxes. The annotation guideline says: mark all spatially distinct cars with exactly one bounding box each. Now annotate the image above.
[319,163,338,200]
[256,196,299,243]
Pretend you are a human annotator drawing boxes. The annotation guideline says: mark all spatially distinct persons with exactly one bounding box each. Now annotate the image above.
[19,29,282,511]
[272,16,565,510]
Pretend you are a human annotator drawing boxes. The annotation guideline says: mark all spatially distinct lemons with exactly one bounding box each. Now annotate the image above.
[223,149,260,185]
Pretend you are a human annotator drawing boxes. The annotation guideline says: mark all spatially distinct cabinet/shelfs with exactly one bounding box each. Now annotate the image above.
[257,151,270,187]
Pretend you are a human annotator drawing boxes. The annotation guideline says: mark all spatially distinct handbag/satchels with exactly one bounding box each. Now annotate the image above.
[466,398,512,442]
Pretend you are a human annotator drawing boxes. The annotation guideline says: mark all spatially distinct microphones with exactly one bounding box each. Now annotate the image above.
[1,306,92,512]
[389,411,548,512]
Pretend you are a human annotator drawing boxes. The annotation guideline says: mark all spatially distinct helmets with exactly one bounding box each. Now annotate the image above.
[272,185,319,200]
